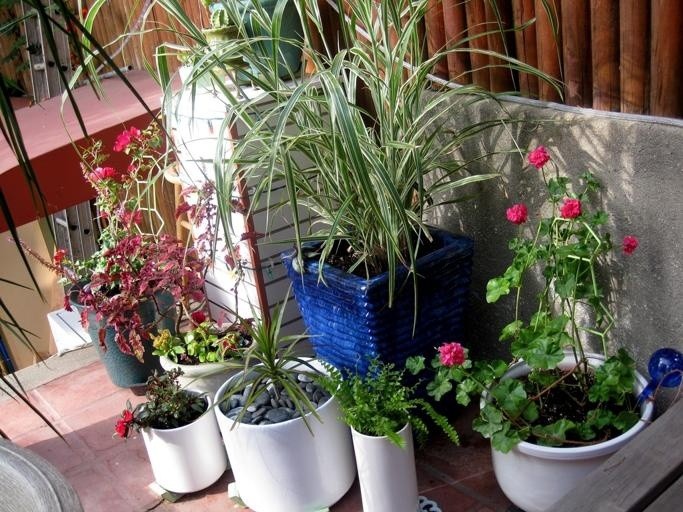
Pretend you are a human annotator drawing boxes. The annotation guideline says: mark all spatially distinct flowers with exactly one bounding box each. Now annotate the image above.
[436,146,655,436]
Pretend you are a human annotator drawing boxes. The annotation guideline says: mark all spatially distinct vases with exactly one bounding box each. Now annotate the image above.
[141,392,231,500]
[481,352,655,511]
